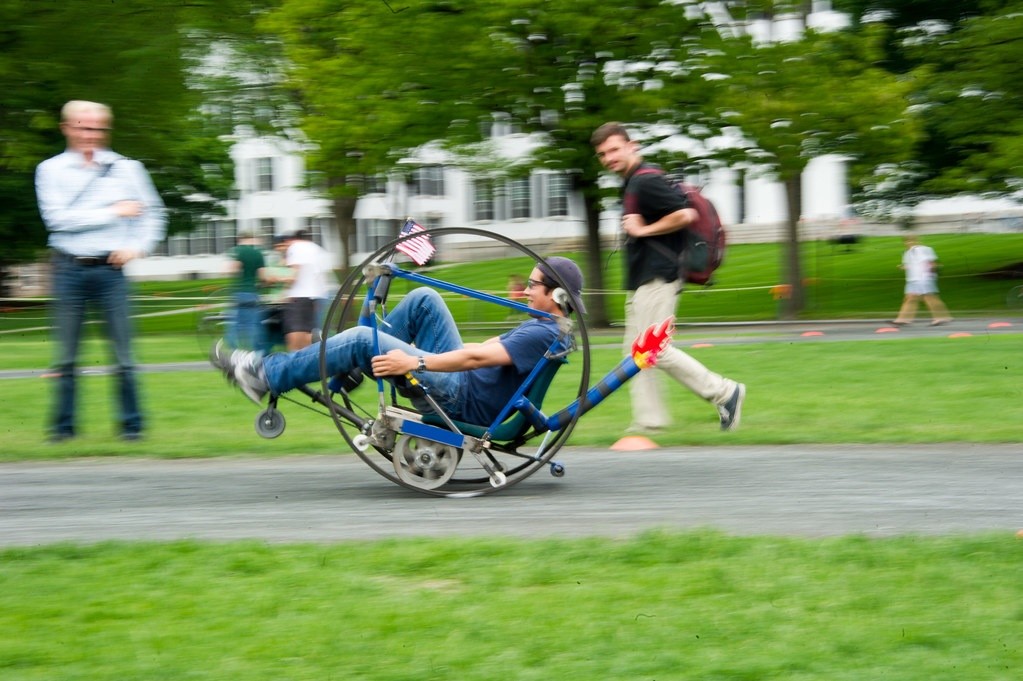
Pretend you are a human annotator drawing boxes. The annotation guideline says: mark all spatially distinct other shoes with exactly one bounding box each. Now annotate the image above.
[928,316,953,327]
[117,425,138,441]
[46,428,73,446]
[886,319,912,327]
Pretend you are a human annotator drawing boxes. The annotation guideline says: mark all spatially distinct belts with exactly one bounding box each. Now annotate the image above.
[69,255,108,268]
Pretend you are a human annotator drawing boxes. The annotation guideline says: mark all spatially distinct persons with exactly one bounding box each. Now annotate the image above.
[890,232,949,327]
[215,254,588,424]
[591,123,746,432]
[224,233,332,355]
[36,99,166,441]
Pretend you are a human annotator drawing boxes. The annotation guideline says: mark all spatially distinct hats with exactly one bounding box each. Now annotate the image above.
[538,256,588,315]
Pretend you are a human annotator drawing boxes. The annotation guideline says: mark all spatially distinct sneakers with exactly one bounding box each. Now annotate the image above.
[717,383,744,432]
[211,337,270,405]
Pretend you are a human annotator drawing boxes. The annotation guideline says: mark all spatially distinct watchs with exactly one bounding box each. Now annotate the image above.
[416,356,426,374]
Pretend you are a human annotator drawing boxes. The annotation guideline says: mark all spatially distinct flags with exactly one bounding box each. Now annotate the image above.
[396,218,437,265]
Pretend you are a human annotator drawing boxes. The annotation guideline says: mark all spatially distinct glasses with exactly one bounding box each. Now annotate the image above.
[527,278,545,289]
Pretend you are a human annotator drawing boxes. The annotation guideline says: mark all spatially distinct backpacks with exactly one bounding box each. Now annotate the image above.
[622,171,725,285]
